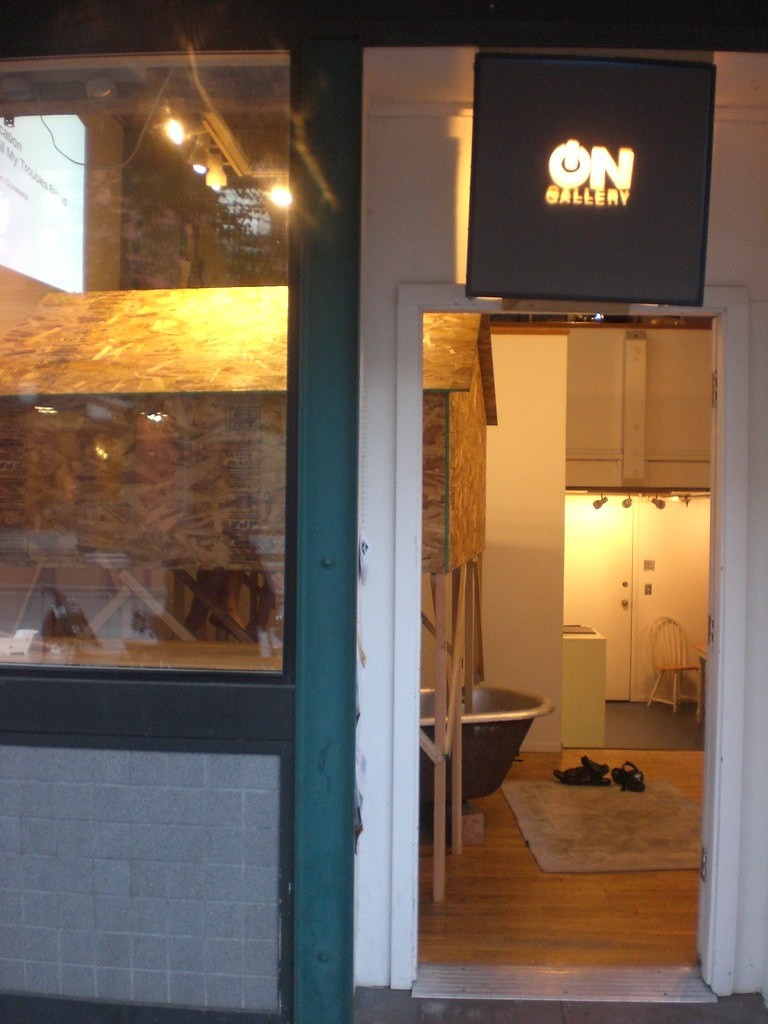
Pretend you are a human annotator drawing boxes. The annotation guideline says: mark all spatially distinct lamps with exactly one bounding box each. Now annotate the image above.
[205,155,227,189]
[168,115,207,144]
[652,493,665,509]
[593,491,607,509]
[190,136,215,173]
[623,493,633,509]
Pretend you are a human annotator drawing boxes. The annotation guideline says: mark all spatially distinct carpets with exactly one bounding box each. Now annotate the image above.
[502,778,703,870]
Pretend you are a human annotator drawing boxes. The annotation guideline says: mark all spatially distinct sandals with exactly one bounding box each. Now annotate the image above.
[554,754,612,787]
[610,761,646,792]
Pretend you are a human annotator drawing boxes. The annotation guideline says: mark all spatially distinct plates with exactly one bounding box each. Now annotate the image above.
[84,553,129,569]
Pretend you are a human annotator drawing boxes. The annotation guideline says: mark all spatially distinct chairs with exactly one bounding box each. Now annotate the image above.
[647,614,698,714]
